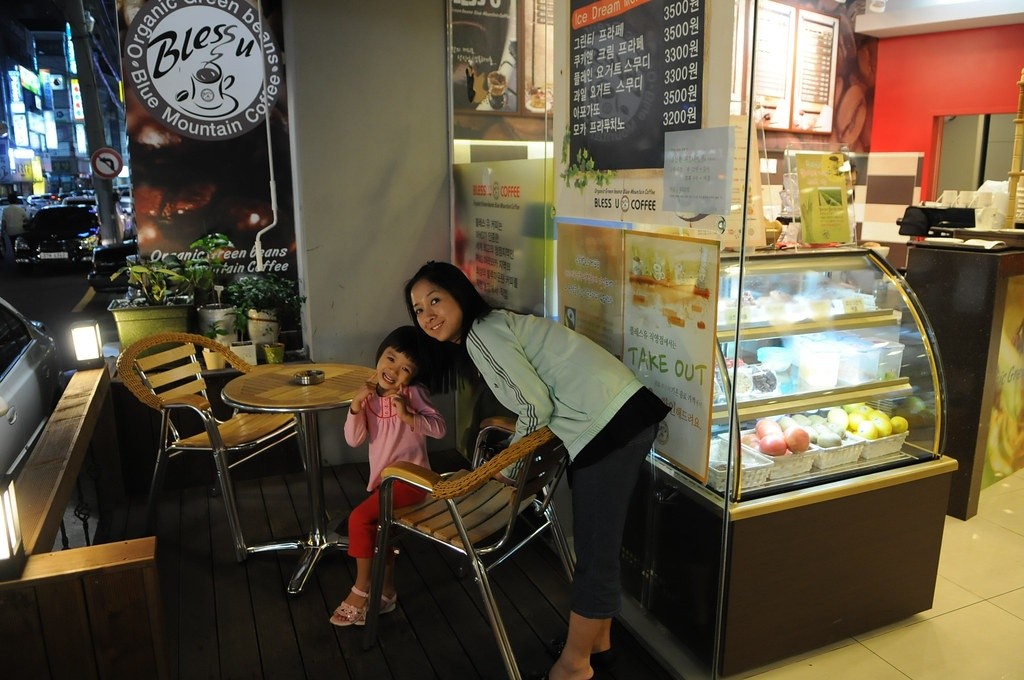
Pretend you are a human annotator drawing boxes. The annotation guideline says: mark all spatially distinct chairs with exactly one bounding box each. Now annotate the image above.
[117,332,307,564]
[363,415,566,680]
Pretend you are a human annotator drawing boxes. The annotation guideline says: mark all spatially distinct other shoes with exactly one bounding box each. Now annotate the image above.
[547,637,614,668]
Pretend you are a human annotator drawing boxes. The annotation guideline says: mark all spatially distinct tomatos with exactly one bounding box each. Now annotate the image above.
[742,417,810,458]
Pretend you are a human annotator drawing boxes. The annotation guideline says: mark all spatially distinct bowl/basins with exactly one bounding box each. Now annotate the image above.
[779,172,801,210]
[756,346,792,370]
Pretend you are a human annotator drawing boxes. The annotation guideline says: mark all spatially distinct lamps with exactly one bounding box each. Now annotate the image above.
[70,319,106,372]
[0,475,27,581]
[870,0,888,13]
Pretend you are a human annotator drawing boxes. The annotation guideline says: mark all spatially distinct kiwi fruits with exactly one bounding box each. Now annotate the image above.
[792,408,850,449]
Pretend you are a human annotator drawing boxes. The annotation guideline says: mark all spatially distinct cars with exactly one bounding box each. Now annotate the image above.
[0,184,135,240]
[15,205,103,269]
[0,294,64,490]
[85,235,139,293]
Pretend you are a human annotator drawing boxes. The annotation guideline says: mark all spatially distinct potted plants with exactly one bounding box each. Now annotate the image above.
[106,255,197,352]
[229,274,305,347]
[199,319,228,371]
[262,327,285,364]
[223,304,258,368]
[184,234,243,339]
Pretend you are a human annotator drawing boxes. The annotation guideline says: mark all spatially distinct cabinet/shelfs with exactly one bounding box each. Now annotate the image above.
[623,248,961,679]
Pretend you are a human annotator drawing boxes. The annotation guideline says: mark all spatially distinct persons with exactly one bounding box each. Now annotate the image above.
[402,258,672,680]
[329,324,449,626]
[1,190,28,255]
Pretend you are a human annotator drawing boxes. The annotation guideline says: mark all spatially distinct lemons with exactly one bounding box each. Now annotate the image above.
[843,402,909,440]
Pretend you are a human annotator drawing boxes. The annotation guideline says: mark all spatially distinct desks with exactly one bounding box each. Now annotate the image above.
[219,362,402,600]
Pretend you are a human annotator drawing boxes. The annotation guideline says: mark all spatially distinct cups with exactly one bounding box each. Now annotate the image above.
[934,188,995,229]
[1015,170,1024,223]
[851,203,863,245]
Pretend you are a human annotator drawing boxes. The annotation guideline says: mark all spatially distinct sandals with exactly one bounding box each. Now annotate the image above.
[355,593,397,626]
[329,586,370,627]
